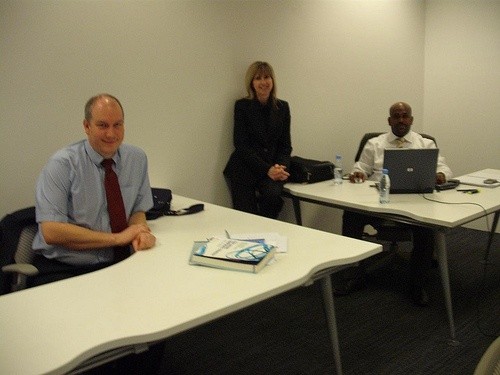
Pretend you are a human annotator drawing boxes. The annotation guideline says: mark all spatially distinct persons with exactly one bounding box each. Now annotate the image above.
[333,102,452,305]
[222,61,293,220]
[26,93,165,375]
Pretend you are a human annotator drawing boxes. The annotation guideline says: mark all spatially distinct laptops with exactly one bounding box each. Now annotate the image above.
[375,148,439,193]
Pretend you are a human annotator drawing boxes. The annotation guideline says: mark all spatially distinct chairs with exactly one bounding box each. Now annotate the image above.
[0,207,43,295]
[341,129,437,264]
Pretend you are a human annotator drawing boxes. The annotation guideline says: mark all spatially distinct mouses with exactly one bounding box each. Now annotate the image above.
[354,177,362,183]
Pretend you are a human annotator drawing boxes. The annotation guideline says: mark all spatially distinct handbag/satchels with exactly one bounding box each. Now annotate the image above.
[288,156,335,183]
[144,188,172,219]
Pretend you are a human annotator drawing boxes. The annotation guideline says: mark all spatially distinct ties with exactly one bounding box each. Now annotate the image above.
[397,137,406,149]
[100,159,131,263]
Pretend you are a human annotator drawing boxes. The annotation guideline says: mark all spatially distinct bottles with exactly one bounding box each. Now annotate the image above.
[334,156,343,184]
[379,169,390,203]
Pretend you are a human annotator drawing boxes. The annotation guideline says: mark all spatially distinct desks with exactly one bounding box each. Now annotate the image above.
[283,169,500,348]
[0,188,383,375]
